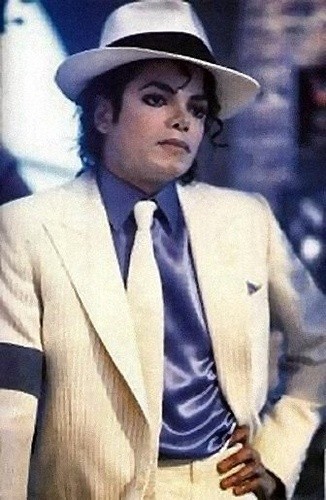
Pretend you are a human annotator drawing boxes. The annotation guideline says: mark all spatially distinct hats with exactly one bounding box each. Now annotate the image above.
[54,0,261,120]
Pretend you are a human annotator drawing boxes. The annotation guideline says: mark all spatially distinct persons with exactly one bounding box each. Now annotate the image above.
[0,2,326,500]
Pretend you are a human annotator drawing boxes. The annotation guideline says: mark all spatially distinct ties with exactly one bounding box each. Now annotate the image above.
[126,200,164,465]
